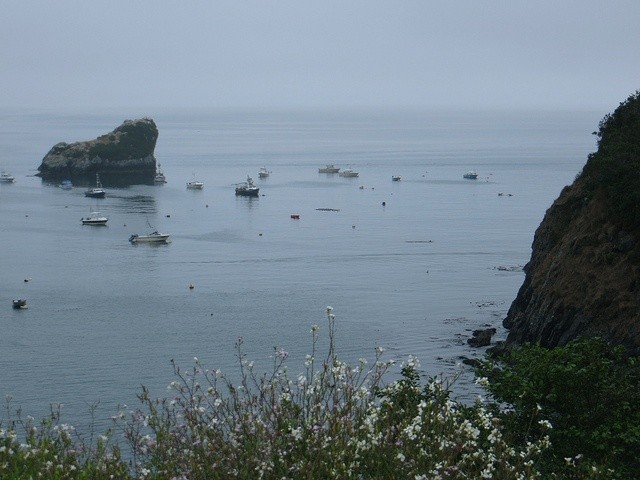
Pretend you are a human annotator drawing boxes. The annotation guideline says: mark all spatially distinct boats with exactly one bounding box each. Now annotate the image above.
[464,171,478,178]
[0,175,14,182]
[186,181,203,189]
[258,168,269,177]
[129,231,169,243]
[80,212,108,225]
[319,165,339,173]
[339,169,359,176]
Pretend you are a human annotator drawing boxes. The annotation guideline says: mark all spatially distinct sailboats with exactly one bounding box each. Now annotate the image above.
[155,163,166,183]
[84,173,105,197]
[235,176,259,196]
